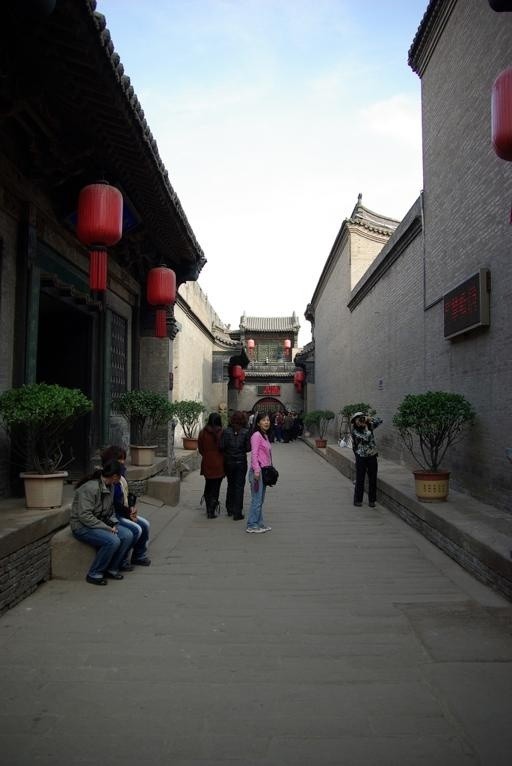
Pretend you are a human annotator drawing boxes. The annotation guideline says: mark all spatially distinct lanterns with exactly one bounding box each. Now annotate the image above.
[294,380,301,393]
[284,337,291,355]
[148,264,176,338]
[78,179,123,303]
[238,372,245,389]
[488,66,511,162]
[248,338,255,353]
[295,370,304,385]
[232,363,241,388]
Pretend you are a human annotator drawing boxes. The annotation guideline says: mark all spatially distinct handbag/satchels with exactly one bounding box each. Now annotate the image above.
[261,465,279,488]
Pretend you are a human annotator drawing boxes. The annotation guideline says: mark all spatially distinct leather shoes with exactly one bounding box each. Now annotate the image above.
[354,502,362,506]
[86,573,107,584]
[369,501,376,506]
[103,569,123,579]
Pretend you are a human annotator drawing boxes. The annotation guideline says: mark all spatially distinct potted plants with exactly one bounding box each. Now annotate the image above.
[0,382,96,510]
[172,399,208,452]
[111,390,175,467]
[300,408,336,450]
[392,389,479,506]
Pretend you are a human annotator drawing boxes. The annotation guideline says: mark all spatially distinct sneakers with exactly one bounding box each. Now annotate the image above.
[138,558,151,565]
[262,526,272,530]
[122,561,134,570]
[245,526,266,533]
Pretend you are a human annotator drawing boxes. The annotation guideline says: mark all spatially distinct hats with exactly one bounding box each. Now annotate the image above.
[350,411,364,423]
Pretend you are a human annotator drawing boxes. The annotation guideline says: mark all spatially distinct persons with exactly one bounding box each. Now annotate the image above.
[102,446,151,567]
[245,414,275,534]
[196,414,228,518]
[236,404,308,443]
[70,462,132,587]
[221,409,248,521]
[350,413,383,508]
